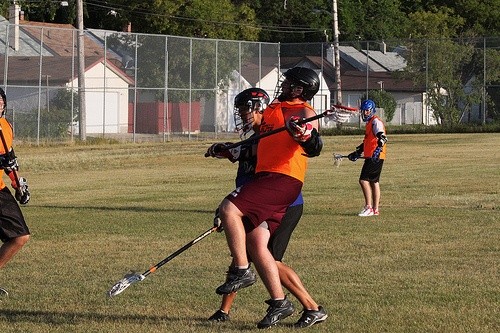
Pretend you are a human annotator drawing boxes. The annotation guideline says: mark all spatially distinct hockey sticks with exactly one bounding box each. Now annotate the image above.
[331,153,381,169]
[204,104,359,157]
[107,227,217,299]
[0,123,24,197]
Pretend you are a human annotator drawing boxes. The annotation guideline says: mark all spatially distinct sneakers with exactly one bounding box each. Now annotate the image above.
[359,208,373,216]
[216,263,257,294]
[372,208,379,215]
[294,305,328,329]
[207,310,230,322]
[257,295,294,328]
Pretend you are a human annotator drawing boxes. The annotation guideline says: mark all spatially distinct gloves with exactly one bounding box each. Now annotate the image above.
[286,116,313,141]
[214,207,223,232]
[207,142,241,163]
[372,147,382,162]
[348,150,361,161]
[0,153,19,171]
[11,177,30,204]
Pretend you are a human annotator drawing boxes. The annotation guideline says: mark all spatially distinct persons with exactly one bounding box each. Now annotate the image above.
[215,66,326,326]
[204,88,330,328]
[347,100,387,216]
[0,89,31,299]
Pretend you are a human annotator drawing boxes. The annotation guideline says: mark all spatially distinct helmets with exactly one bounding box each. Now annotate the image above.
[360,100,375,122]
[234,88,270,113]
[273,67,320,100]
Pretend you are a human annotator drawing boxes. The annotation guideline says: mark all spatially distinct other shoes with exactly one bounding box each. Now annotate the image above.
[0,289,8,296]
